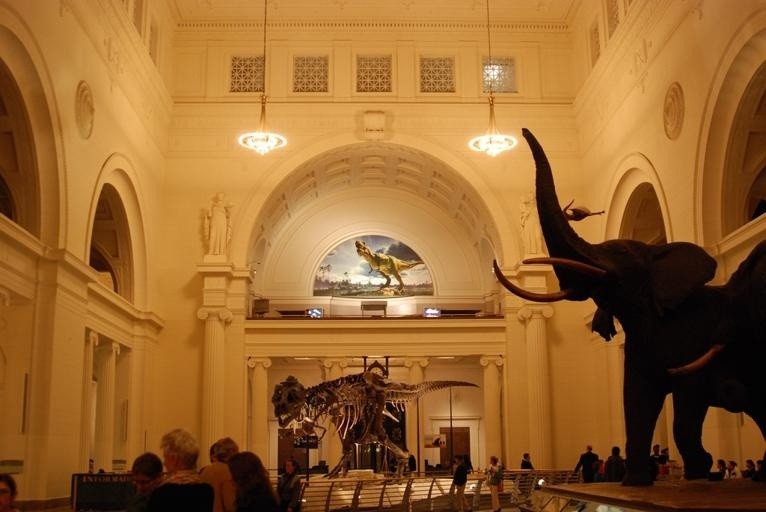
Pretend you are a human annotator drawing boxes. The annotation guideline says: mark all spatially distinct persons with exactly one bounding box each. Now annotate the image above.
[130,427,301,512]
[573,444,763,483]
[452,453,473,512]
[521,452,536,476]
[0,472,21,512]
[486,455,503,512]
[463,454,474,474]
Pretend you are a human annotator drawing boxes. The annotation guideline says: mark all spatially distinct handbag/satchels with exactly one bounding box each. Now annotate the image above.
[490,467,503,486]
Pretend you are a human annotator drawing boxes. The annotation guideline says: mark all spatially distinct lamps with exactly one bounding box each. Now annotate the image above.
[466,0,517,157]
[237,0,287,155]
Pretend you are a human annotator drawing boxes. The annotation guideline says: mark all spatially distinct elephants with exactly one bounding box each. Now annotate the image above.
[665,235,766,484]
[488,124,728,491]
